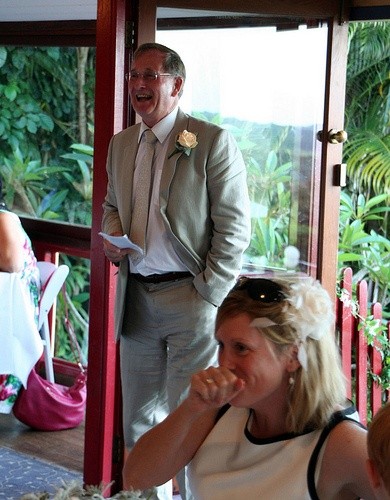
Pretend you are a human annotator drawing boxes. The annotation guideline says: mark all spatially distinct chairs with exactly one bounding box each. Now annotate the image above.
[34,259,70,385]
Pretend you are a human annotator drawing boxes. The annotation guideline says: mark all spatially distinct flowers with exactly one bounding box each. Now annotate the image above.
[167,128,201,160]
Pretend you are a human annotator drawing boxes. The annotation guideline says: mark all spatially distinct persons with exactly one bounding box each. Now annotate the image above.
[364,400,390,500]
[102,43,251,500]
[123,273,390,500]
[0,180,41,414]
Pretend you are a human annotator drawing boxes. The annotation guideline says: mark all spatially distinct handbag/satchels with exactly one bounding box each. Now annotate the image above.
[11,355,89,431]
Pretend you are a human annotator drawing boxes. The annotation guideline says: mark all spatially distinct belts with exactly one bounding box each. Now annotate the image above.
[130,270,195,284]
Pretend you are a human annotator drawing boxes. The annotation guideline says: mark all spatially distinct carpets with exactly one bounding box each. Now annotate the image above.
[0,442,84,500]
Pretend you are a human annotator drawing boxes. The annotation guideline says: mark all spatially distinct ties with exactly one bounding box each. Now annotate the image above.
[128,129,158,266]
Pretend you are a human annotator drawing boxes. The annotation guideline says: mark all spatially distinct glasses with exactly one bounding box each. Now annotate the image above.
[125,69,178,82]
[229,277,292,304]
